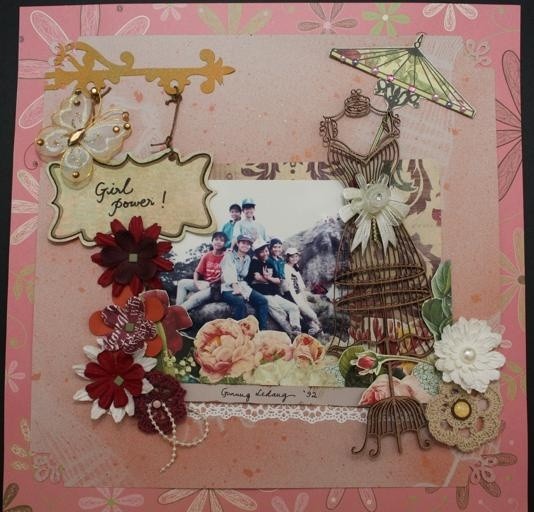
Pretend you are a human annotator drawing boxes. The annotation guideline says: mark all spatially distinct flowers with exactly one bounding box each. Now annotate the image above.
[425,382,502,453]
[75,216,193,430]
[350,351,433,376]
[433,314,505,394]
[192,314,341,389]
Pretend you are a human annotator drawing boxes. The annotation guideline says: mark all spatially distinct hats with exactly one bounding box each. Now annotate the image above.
[251,238,270,253]
[237,234,253,244]
[285,247,301,256]
[241,197,256,207]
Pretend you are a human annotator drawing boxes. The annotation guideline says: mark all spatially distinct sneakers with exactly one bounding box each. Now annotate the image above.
[307,321,323,336]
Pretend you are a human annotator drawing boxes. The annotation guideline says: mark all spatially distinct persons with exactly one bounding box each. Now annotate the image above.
[175,231,227,310]
[247,239,302,344]
[222,204,241,246]
[262,238,295,303]
[232,197,263,249]
[282,247,321,341]
[219,234,268,331]
